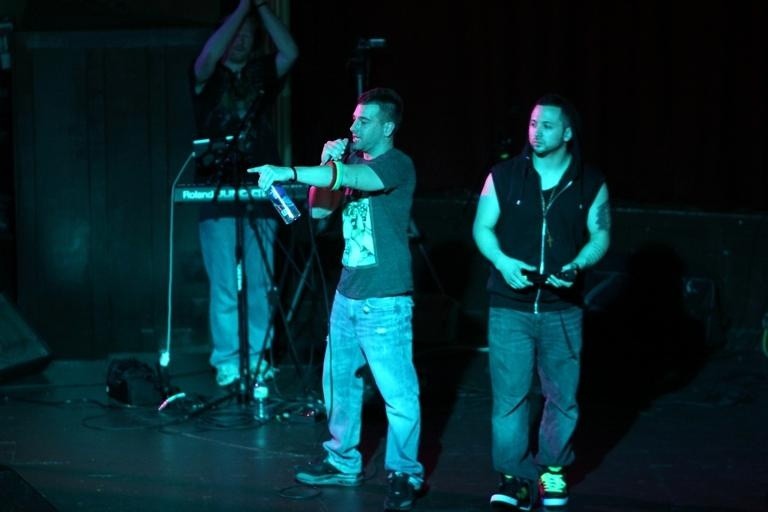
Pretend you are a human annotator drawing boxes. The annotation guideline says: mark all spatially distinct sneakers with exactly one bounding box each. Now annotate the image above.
[537,465,569,507]
[215,359,241,387]
[382,471,416,511]
[295,449,365,487]
[244,353,279,382]
[489,472,534,512]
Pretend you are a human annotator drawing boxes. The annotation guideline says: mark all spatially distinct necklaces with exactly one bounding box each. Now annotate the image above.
[539,159,559,248]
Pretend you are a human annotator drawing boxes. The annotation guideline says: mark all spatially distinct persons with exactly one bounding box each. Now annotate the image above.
[186,0,300,391]
[247,85,428,512]
[471,94,614,510]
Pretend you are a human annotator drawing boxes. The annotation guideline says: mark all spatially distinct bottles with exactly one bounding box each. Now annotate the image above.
[265,182,301,224]
[250,375,272,425]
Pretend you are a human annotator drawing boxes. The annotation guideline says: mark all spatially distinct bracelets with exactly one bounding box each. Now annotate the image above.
[289,166,298,184]
[254,0,269,10]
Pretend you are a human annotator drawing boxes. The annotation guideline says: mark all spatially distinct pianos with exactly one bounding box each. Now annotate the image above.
[172,181,308,203]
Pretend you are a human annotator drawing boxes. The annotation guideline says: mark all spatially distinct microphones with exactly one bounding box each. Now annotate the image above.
[322,146,349,164]
[523,271,575,282]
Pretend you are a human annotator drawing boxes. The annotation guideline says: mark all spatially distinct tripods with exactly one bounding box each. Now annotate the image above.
[286,72,446,326]
[186,160,324,423]
